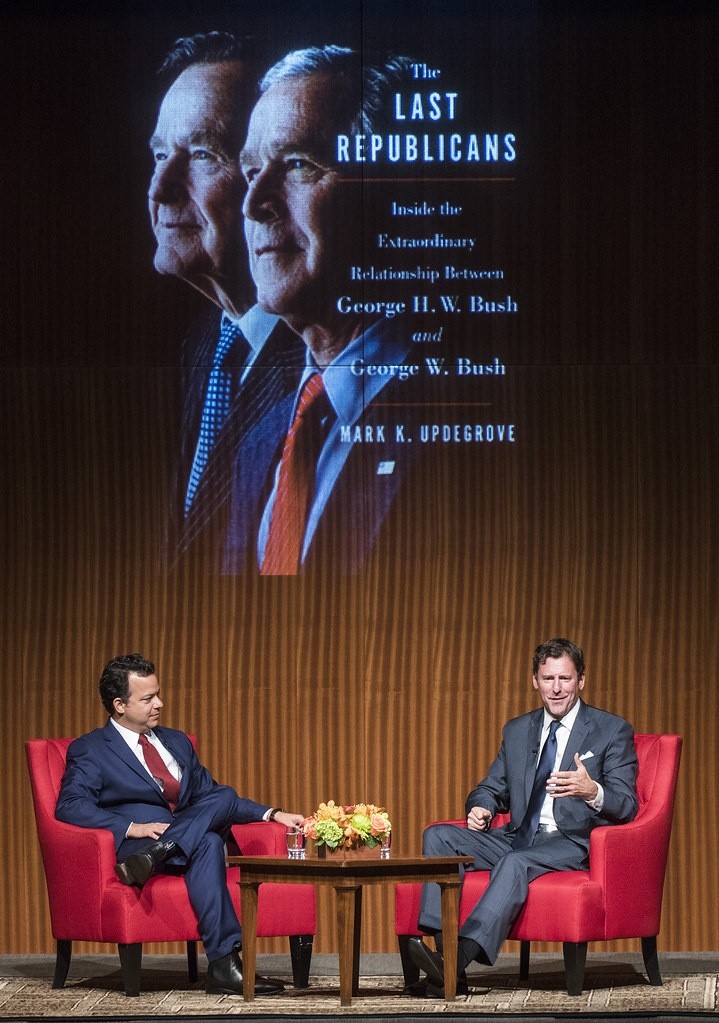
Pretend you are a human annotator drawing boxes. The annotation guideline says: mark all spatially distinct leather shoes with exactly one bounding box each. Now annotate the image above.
[114,841,168,887]
[402,975,469,996]
[206,951,287,996]
[409,935,460,996]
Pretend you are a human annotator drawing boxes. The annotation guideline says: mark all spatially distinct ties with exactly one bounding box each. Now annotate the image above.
[183,321,253,517]
[138,734,181,811]
[510,720,562,852]
[260,373,331,577]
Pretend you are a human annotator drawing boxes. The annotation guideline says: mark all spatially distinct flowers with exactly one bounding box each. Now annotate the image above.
[298,802,391,854]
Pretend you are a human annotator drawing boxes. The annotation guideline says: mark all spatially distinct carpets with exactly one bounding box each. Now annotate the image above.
[0,971,719,1022]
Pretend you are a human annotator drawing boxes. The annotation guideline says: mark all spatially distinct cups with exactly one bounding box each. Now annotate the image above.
[380,828,392,852]
[286,826,306,855]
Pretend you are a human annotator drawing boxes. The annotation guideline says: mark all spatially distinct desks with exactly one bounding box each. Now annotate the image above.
[225,852,474,1007]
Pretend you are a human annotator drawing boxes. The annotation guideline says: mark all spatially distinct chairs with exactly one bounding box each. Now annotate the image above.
[26,734,320,997]
[395,733,685,995]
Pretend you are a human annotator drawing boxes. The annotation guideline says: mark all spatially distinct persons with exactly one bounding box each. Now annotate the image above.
[144,32,452,579]
[403,638,638,998]
[54,654,305,995]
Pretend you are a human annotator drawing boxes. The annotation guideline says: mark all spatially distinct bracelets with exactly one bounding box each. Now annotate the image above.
[269,808,286,822]
